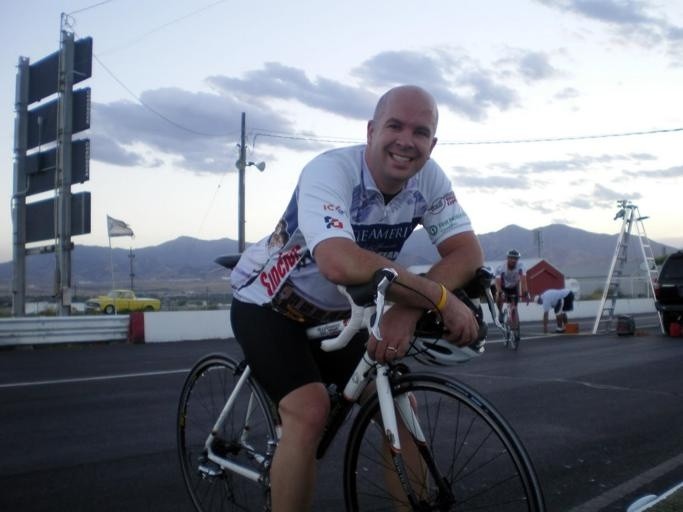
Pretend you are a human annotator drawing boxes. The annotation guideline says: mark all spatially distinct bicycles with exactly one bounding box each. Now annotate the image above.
[176,256,547,512]
[498,291,529,350]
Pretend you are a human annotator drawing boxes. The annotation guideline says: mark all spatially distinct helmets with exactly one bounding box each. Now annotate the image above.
[408,288,489,368]
[506,249,521,258]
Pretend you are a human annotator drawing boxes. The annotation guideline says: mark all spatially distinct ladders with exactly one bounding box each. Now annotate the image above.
[591,205,666,337]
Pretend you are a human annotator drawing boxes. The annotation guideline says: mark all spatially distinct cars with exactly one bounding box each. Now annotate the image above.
[653,251,682,337]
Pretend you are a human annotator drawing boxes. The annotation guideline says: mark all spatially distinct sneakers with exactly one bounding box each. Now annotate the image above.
[555,325,568,332]
[498,312,504,324]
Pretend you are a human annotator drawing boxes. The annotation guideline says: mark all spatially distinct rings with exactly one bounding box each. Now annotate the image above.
[387,346,394,350]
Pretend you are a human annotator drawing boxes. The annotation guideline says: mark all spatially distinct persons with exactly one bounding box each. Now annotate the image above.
[227,82,488,509]
[533,288,575,335]
[493,248,531,344]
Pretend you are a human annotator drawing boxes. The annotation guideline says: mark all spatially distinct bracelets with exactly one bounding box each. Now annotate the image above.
[430,282,447,311]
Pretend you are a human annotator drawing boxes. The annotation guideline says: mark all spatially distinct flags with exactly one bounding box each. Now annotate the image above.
[106,214,133,238]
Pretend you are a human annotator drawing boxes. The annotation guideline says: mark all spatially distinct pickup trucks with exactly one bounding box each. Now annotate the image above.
[84,289,161,316]
